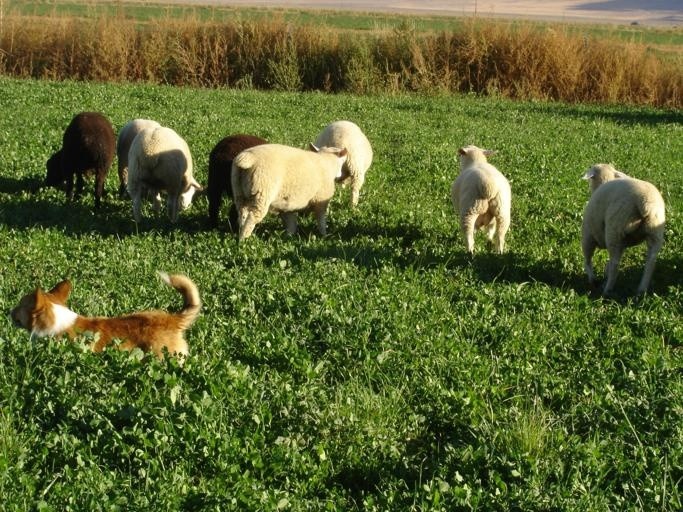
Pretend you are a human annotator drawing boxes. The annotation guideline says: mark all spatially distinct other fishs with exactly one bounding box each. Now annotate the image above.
[46,112,373,242]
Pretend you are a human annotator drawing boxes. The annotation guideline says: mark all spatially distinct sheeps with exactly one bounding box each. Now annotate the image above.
[579,163,666,304]
[451,145,511,259]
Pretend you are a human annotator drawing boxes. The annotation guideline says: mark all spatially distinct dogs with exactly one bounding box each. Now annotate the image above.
[10,270,201,373]
